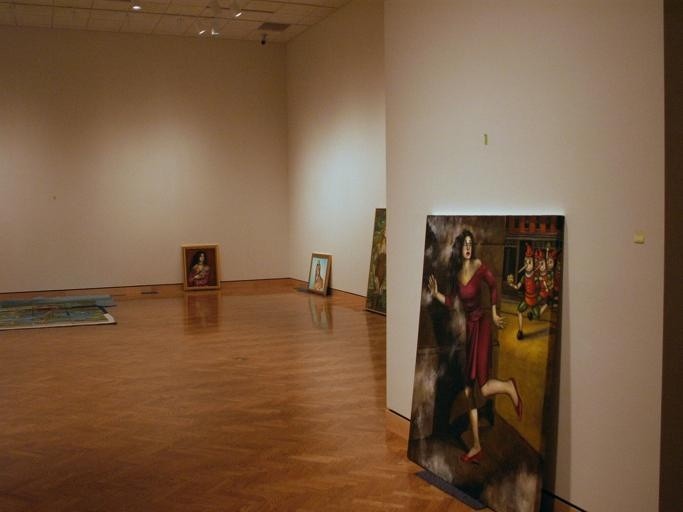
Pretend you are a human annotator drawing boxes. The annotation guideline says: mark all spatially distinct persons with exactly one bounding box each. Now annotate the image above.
[507,243,556,340]
[426,230,523,462]
[188,252,209,287]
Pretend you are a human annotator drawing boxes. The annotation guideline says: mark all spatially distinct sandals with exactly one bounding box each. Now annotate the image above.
[460,446,486,463]
[506,377,524,418]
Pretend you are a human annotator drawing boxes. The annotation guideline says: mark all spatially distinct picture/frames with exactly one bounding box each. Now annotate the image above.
[306,251,332,297]
[179,243,221,292]
